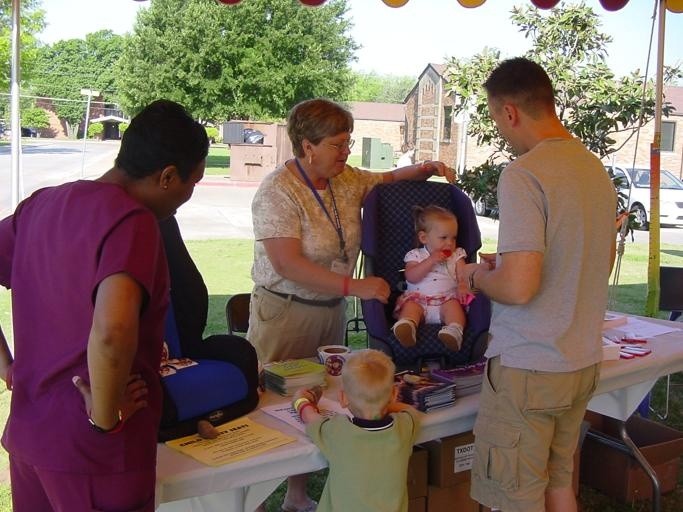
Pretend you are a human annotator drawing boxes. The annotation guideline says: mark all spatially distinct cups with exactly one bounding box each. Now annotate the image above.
[316,345,350,379]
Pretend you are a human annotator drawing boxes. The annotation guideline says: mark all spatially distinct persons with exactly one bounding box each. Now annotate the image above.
[394,201,471,353]
[1,99,214,512]
[244,95,457,512]
[292,347,424,512]
[464,57,622,512]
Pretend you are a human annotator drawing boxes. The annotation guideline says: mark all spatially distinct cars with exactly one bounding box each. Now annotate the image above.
[473,160,510,216]
[603,164,683,231]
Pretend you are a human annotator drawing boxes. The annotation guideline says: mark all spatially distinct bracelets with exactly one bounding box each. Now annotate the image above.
[5,363,15,391]
[469,267,480,292]
[87,405,126,434]
[343,275,350,296]
[294,396,311,411]
[420,159,434,175]
[298,402,320,423]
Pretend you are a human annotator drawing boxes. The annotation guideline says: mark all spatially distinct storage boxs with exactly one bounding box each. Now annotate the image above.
[407,430,492,512]
[579,411,683,502]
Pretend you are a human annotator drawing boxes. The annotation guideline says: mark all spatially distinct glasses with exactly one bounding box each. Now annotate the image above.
[323,139,355,150]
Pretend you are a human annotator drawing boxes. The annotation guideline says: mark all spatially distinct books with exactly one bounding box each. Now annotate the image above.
[262,358,328,398]
[393,360,487,414]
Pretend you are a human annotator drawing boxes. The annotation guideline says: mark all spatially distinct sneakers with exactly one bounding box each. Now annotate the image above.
[438,326,462,352]
[390,320,417,347]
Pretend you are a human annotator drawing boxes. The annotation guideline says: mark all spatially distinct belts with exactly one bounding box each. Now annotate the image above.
[262,284,344,306]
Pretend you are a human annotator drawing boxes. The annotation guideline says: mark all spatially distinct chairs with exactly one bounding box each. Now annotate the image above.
[226,293,251,337]
[362,180,491,371]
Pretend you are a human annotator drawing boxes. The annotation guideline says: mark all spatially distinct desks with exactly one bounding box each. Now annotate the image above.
[155,308,682,512]
